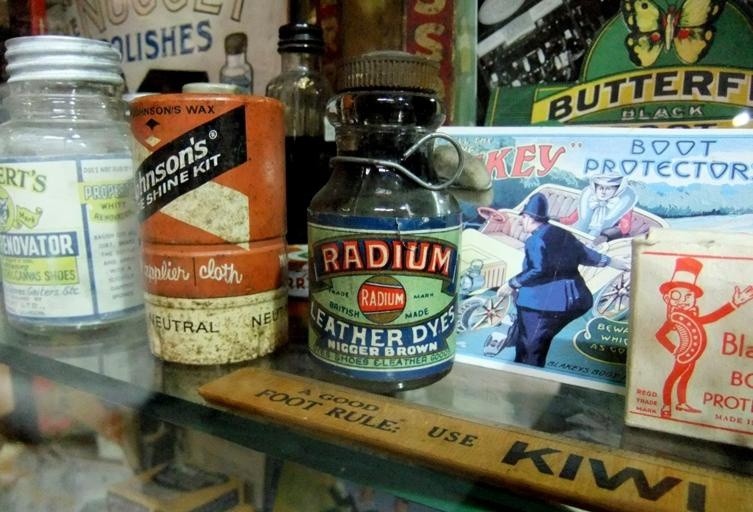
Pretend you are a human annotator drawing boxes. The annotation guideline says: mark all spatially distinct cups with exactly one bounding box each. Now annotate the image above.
[131,94,290,367]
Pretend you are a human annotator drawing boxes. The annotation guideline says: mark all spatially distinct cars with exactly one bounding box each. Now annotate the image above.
[455,183,670,333]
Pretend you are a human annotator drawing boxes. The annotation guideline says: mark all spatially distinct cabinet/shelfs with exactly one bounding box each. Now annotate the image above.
[0,302,751,512]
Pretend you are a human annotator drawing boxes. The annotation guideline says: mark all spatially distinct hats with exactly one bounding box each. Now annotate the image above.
[592,172,624,186]
[659,257,704,297]
[518,192,549,220]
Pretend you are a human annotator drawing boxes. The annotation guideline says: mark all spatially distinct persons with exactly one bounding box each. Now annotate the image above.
[497,192,627,366]
[550,171,636,247]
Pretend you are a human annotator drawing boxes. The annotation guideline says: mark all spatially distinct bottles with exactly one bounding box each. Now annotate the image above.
[0,34,142,336]
[261,18,331,324]
[306,45,456,389]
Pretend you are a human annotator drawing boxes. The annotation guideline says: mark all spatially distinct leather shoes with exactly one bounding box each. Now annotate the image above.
[660,404,672,419]
[676,402,702,413]
